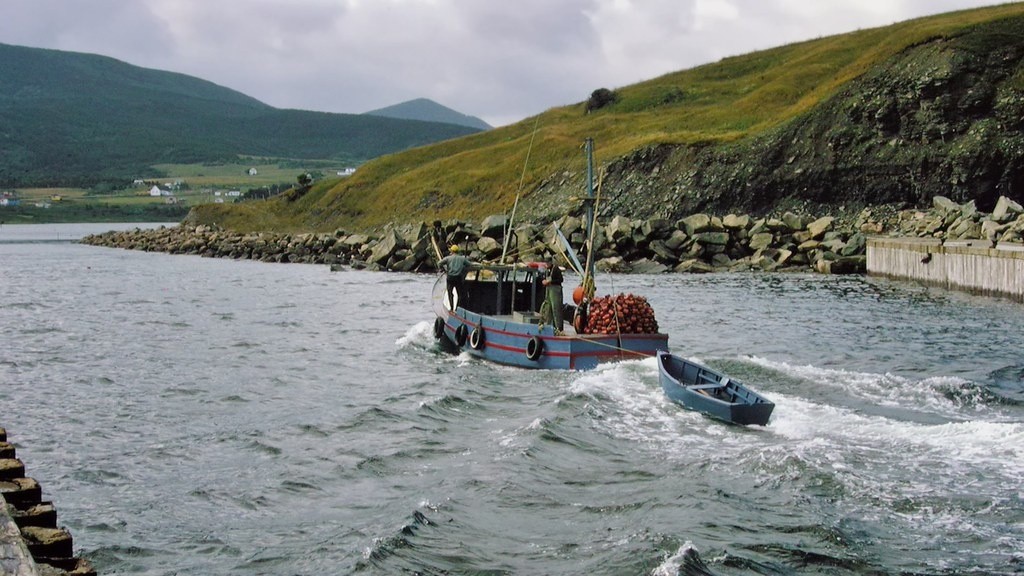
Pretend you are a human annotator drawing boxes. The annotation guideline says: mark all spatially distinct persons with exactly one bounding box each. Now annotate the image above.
[437,245,472,312]
[538,256,564,332]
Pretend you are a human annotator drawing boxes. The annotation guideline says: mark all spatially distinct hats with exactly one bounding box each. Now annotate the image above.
[543,256,552,262]
[450,245,459,251]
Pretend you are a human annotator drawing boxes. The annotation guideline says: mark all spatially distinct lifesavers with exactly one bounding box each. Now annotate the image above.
[454,325,467,347]
[469,326,484,350]
[433,317,444,338]
[525,336,542,361]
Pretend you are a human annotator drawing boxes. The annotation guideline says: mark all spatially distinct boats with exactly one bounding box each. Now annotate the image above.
[429,112,669,375]
[656,346,776,428]
